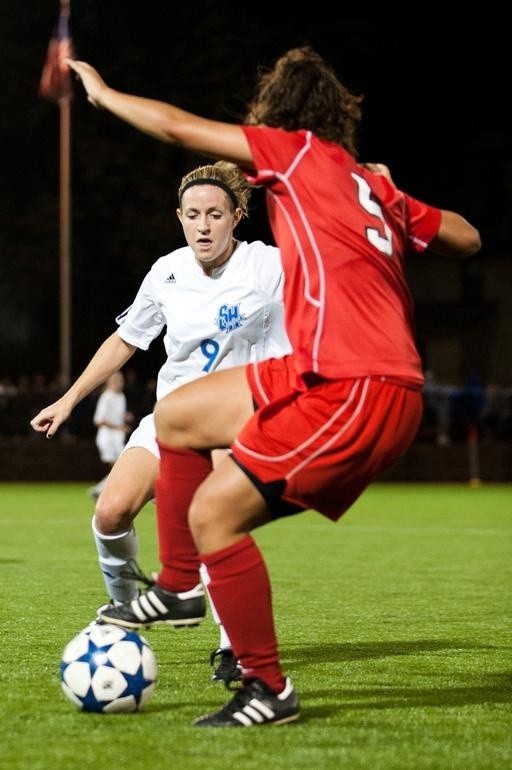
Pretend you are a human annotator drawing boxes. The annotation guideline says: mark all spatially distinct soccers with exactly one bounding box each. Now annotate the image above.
[60,624,158,712]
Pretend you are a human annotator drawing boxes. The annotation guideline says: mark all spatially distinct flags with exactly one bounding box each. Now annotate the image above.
[39,1,74,105]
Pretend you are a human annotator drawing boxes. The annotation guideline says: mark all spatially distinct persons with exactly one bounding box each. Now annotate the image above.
[63,47,486,726]
[87,370,135,506]
[30,159,293,682]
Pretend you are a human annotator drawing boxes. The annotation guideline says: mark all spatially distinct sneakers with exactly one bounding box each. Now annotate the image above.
[96,582,207,630]
[191,676,301,729]
[211,648,242,681]
